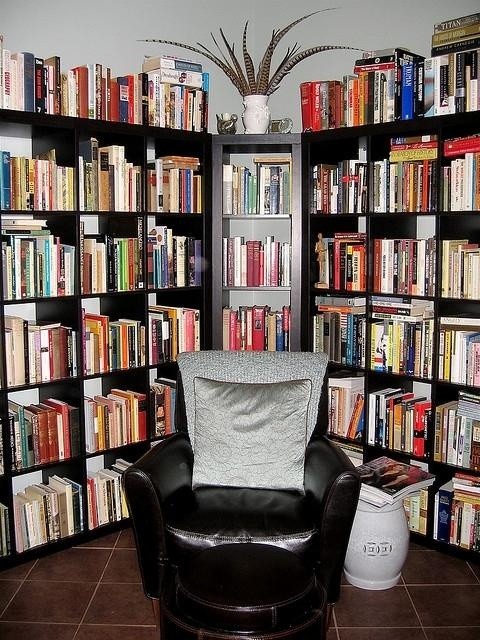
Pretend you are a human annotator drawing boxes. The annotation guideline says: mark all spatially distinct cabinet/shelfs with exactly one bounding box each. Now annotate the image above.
[0,107,480,572]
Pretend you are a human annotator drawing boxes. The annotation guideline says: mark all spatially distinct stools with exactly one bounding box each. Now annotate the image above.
[157,541,328,640]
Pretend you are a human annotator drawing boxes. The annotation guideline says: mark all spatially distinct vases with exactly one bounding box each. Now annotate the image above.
[242,94,271,134]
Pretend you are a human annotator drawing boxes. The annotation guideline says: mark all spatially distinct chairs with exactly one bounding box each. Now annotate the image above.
[118,349,363,632]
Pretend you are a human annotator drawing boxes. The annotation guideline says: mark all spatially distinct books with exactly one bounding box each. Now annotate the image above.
[223,157,291,353]
[1,49,209,133]
[1,138,201,470]
[300,11,480,132]
[311,132,480,553]
[1,459,131,556]
